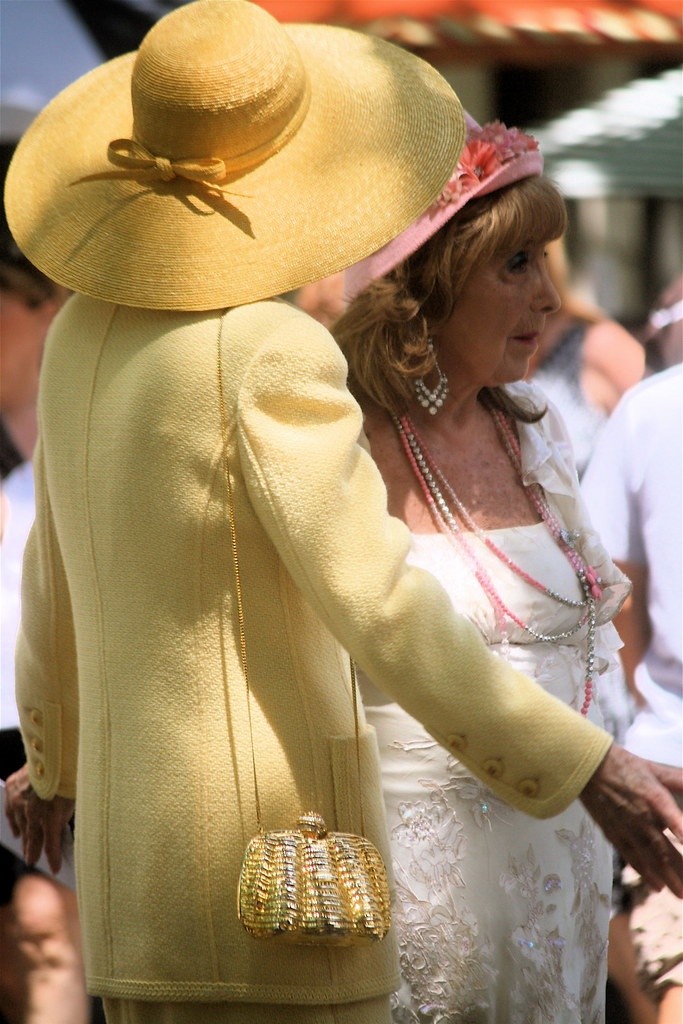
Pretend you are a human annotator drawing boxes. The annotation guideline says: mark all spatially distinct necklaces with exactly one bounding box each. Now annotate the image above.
[395,407,603,715]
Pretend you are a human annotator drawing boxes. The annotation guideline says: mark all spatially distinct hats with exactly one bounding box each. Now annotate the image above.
[4,0,466,311]
[342,107,543,317]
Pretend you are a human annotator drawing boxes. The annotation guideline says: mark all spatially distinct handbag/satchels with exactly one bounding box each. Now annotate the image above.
[237,814,391,949]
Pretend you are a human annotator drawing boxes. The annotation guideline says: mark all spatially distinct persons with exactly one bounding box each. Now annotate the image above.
[0,128,94,1024]
[522,228,683,1006]
[296,116,632,1024]
[2,0,683,1024]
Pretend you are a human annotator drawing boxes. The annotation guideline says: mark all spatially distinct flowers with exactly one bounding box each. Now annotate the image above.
[439,118,539,205]
